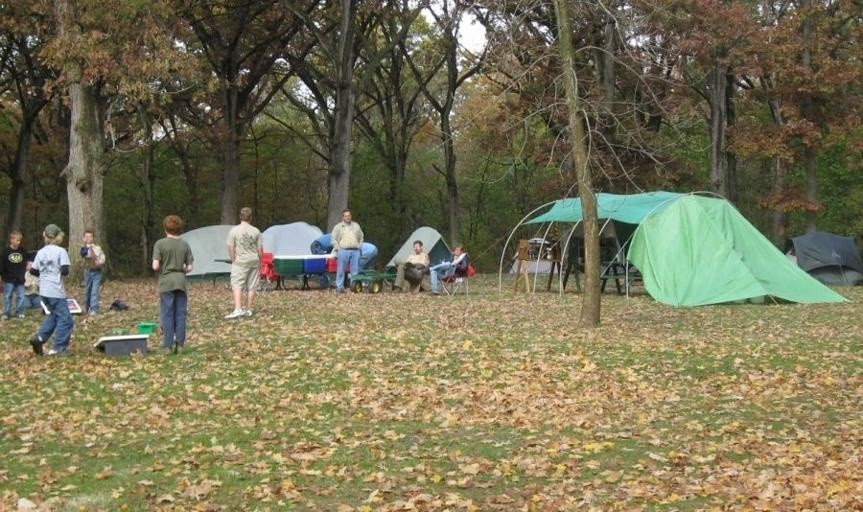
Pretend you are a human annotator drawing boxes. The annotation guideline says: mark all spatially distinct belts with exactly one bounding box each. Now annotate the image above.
[345,248,358,250]
[86,268,101,272]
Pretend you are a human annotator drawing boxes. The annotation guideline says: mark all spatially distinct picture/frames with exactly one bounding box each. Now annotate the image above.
[39,298,82,316]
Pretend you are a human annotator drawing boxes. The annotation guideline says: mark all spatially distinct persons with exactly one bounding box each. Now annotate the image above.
[23,260,40,309]
[30,223,72,357]
[0,230,28,320]
[331,209,364,293]
[152,215,194,355]
[392,240,430,294]
[429,244,469,295]
[225,207,264,319]
[80,230,105,316]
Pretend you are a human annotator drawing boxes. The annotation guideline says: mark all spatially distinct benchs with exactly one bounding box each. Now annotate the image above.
[601,274,642,282]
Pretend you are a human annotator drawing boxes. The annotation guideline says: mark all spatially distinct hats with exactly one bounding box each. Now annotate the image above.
[44,224,60,239]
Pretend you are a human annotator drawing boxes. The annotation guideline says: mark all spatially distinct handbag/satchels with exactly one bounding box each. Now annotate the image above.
[110,296,128,310]
[466,255,477,276]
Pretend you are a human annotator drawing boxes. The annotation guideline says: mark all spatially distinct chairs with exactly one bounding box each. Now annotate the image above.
[440,263,469,298]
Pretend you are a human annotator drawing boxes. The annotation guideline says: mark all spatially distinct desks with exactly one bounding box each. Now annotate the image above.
[601,260,642,296]
[274,272,337,290]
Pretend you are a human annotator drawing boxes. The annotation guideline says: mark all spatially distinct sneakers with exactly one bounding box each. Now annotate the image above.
[391,287,402,293]
[405,262,416,269]
[428,291,441,297]
[243,306,253,318]
[16,313,25,319]
[225,311,246,320]
[48,348,74,356]
[3,314,9,321]
[30,335,44,355]
[420,268,430,275]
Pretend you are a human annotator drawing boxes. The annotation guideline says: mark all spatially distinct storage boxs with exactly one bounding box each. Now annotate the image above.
[272,255,304,275]
[137,323,156,335]
[304,254,327,274]
[327,254,351,273]
[93,334,149,356]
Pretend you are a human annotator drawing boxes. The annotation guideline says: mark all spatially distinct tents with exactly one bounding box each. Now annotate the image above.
[784,230,863,285]
[385,225,452,269]
[510,238,558,274]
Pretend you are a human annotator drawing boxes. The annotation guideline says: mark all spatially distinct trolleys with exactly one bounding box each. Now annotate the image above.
[350,268,397,295]
